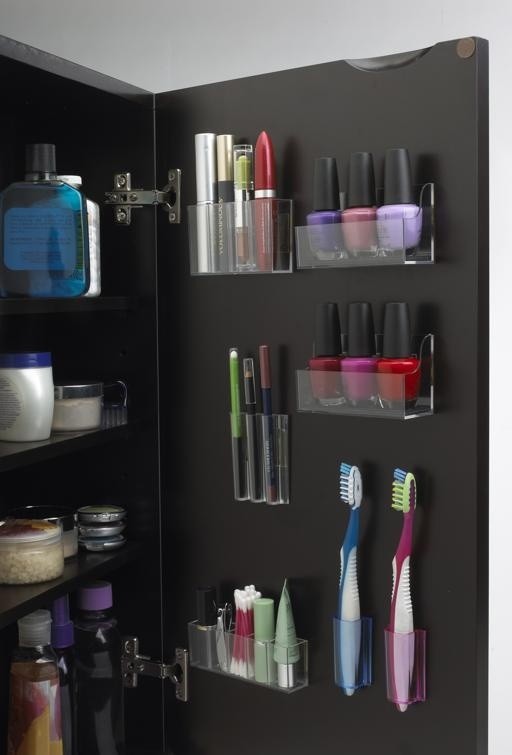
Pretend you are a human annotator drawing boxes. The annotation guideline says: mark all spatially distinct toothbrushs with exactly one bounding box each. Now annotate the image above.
[390,469,416,716]
[339,461,363,697]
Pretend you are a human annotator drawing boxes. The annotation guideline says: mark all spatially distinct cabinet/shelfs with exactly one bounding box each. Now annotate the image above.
[296,184,435,271]
[383,623,426,709]
[189,622,310,696]
[296,328,435,420]
[187,190,295,278]
[2,88,157,750]
[234,412,289,505]
[332,612,373,696]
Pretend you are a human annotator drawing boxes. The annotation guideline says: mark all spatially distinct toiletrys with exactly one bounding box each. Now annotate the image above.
[272,580,302,689]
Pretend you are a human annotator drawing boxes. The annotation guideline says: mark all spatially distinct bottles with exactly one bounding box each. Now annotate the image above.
[0,519,64,585]
[73,581,126,755]
[7,609,63,755]
[6,505,78,559]
